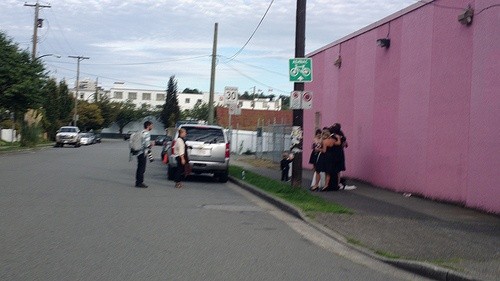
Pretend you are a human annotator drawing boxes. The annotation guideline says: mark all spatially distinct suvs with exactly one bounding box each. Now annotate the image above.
[54,126,81,148]
[166,123,232,183]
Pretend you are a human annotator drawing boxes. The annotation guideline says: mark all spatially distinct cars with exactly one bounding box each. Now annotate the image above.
[155,136,168,146]
[81,133,102,145]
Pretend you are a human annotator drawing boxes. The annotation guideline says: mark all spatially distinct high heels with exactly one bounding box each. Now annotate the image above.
[310,186,319,191]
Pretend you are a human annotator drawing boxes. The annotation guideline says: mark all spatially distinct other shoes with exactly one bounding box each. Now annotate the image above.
[135,182,148,188]
[175,183,183,189]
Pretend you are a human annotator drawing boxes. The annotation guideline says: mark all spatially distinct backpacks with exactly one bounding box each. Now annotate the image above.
[129,130,147,152]
[171,139,184,156]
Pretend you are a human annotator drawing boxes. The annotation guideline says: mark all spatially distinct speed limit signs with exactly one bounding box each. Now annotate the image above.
[224,86,239,109]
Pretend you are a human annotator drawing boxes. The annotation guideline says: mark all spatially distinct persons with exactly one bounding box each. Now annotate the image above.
[288,136,294,177]
[135,120,155,188]
[309,123,348,192]
[280,153,294,181]
[172,128,187,188]
